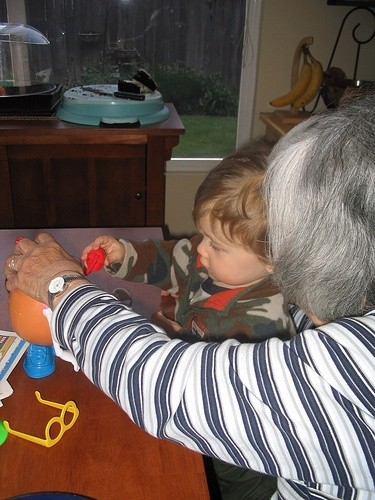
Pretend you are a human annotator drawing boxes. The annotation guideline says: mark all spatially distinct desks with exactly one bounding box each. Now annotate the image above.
[0,225,211,500]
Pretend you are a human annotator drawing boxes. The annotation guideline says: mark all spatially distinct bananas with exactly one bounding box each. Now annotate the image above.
[269,44,325,109]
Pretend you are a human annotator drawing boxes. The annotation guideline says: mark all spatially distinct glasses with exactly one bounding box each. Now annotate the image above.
[4,391,80,448]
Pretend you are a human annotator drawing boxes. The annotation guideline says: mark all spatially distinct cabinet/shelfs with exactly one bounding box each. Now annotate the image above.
[0,102,186,229]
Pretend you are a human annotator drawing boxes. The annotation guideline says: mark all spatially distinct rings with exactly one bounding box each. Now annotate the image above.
[8,236,25,274]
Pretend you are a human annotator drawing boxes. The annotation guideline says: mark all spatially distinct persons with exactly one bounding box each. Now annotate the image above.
[80,145,298,500]
[4,93,375,500]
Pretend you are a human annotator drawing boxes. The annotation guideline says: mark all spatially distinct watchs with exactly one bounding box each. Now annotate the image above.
[48,274,88,312]
